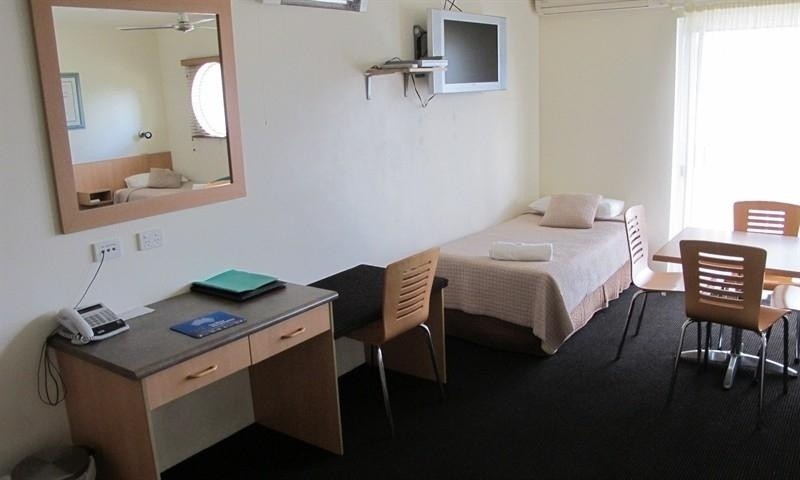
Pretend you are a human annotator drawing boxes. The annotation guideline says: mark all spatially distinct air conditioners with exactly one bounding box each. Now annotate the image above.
[534,0,650,15]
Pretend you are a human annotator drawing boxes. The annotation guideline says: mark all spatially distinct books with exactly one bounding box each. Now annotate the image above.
[190,269,287,303]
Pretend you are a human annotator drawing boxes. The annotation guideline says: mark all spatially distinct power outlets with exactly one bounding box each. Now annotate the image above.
[137,230,165,251]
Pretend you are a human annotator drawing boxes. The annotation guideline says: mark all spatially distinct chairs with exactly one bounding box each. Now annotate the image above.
[670,240,791,416]
[345,246,439,442]
[733,200,800,353]
[614,205,723,362]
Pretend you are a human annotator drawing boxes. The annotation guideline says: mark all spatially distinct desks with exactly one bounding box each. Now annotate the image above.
[652,227,800,391]
[48,274,345,480]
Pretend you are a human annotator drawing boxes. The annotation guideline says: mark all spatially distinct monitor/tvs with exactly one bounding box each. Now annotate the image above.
[429,8,510,94]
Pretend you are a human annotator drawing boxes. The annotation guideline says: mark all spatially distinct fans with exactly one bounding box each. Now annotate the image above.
[114,12,218,33]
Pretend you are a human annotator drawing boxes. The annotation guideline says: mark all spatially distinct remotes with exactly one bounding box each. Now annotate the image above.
[378,63,418,70]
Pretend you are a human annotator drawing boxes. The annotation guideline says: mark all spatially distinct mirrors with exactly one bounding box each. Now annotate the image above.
[29,2,247,235]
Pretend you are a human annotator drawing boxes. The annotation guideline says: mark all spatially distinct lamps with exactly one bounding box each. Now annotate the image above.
[138,129,152,139]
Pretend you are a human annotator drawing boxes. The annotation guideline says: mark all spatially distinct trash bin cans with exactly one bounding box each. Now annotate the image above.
[4,444,100,480]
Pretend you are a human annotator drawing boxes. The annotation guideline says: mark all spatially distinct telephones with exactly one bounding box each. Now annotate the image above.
[55,302,130,342]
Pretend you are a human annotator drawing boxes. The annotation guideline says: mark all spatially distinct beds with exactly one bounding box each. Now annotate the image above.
[434,192,645,359]
[113,178,231,203]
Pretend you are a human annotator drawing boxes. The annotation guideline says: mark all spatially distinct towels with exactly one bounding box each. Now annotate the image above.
[488,240,552,261]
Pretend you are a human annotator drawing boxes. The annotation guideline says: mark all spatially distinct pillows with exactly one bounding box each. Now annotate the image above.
[525,195,550,215]
[538,192,602,229]
[595,197,626,221]
[307,264,448,341]
[147,167,181,188]
[124,169,190,189]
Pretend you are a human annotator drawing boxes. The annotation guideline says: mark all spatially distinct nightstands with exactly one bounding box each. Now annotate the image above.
[77,188,113,206]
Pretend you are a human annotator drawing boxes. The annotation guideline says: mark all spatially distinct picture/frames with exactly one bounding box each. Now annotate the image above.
[59,72,86,129]
